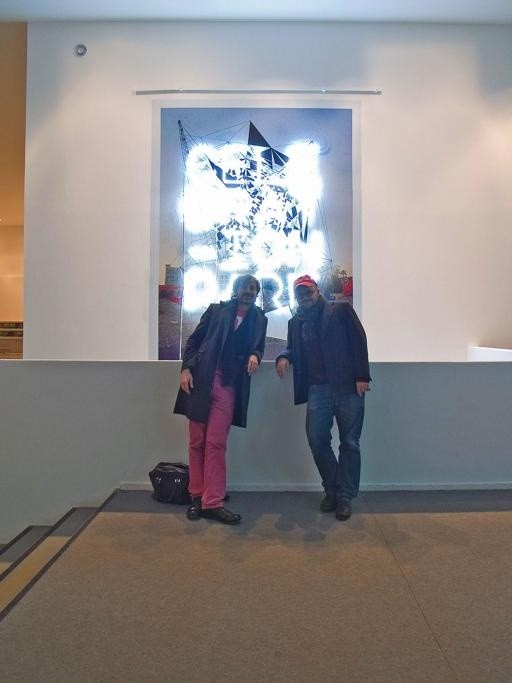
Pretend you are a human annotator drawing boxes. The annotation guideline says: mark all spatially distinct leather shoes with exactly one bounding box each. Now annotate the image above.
[187,496,202,519]
[200,506,241,524]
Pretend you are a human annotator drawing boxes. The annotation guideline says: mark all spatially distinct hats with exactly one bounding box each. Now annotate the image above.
[293,275,316,290]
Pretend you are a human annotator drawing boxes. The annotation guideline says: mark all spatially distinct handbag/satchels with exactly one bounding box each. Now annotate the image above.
[149,462,192,505]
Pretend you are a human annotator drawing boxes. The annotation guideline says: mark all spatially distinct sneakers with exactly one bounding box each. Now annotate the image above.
[320,496,337,511]
[335,500,352,520]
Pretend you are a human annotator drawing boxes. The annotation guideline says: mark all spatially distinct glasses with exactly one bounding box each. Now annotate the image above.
[296,291,315,300]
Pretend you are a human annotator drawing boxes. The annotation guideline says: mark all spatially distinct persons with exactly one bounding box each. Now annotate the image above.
[276,275,372,521]
[172,274,268,525]
